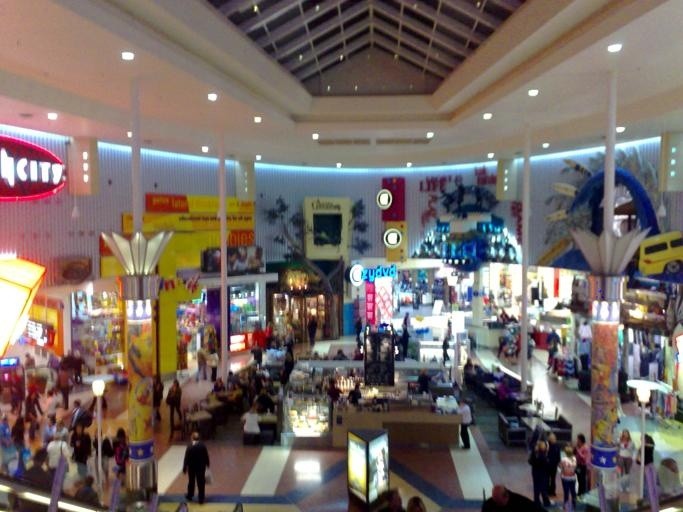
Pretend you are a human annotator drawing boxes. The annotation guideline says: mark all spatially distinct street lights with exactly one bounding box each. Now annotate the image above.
[626,379,671,500]
[92,380,105,506]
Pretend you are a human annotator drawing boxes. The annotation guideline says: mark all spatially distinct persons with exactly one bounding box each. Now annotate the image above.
[498,326,536,360]
[491,368,509,410]
[365,490,425,512]
[483,485,539,510]
[380,326,484,449]
[617,336,660,404]
[152,377,182,426]
[196,314,364,415]
[547,326,592,378]
[183,432,210,504]
[0,351,130,512]
[528,429,660,511]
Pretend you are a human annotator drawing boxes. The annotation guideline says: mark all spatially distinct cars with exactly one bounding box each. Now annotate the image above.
[638,230,683,276]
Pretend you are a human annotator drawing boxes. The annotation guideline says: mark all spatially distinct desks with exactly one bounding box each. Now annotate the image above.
[241,380,280,438]
[521,416,551,434]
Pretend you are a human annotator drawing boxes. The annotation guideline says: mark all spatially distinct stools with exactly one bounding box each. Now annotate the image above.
[243,433,265,446]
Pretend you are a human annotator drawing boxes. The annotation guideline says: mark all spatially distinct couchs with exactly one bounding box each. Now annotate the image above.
[497,411,528,450]
[546,415,572,445]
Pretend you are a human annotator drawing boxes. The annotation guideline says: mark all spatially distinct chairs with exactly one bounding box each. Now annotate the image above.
[169,387,244,441]
